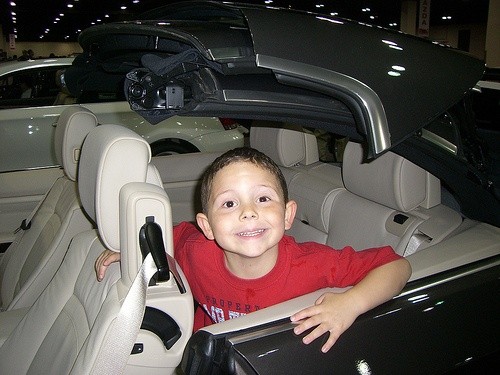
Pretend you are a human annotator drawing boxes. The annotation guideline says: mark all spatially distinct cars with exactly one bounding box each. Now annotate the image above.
[0,54,250,174]
[1,0,500,375]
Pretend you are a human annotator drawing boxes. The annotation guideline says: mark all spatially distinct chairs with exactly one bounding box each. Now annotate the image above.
[0,105,462,375]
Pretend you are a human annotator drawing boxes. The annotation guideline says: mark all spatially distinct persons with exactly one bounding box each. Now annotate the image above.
[0,49,56,63]
[94,147,412,354]
